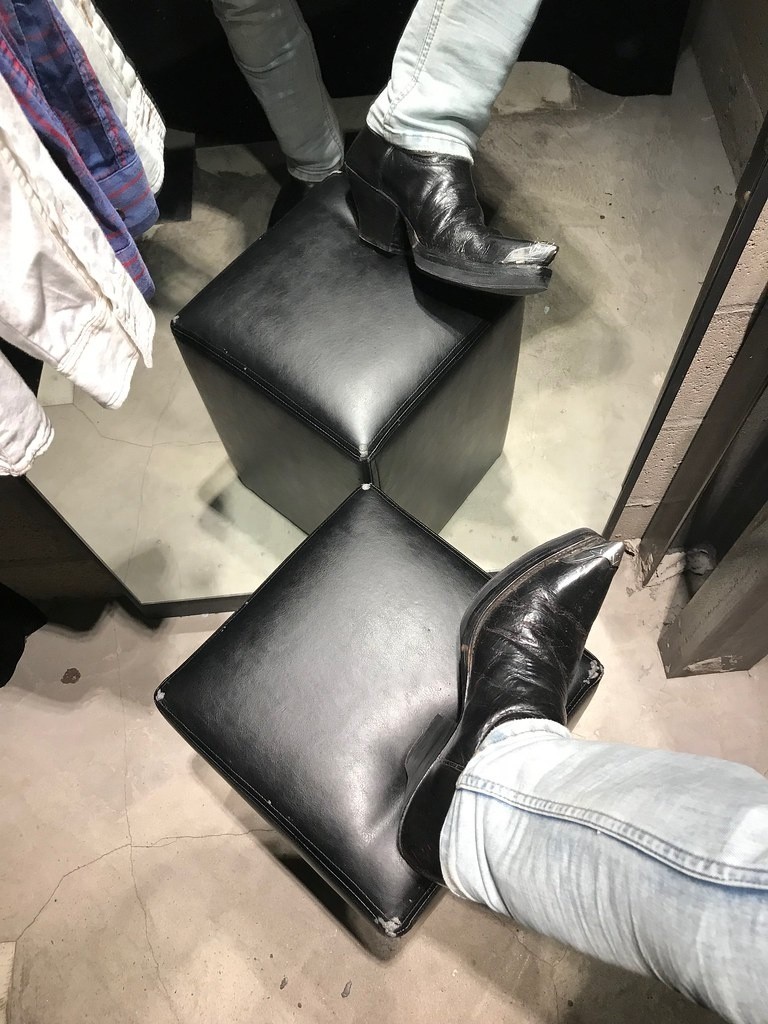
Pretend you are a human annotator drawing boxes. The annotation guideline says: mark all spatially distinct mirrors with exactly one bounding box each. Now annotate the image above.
[0,0,768,620]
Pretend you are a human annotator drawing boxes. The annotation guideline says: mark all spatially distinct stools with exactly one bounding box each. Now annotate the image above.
[151,484,607,968]
[170,170,526,537]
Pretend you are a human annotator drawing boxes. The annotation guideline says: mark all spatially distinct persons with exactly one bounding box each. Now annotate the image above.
[212,0,552,296]
[398,527,767,1024]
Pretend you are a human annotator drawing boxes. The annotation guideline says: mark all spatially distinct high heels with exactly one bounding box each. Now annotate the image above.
[266,170,318,231]
[343,127,559,295]
[396,529,627,888]
